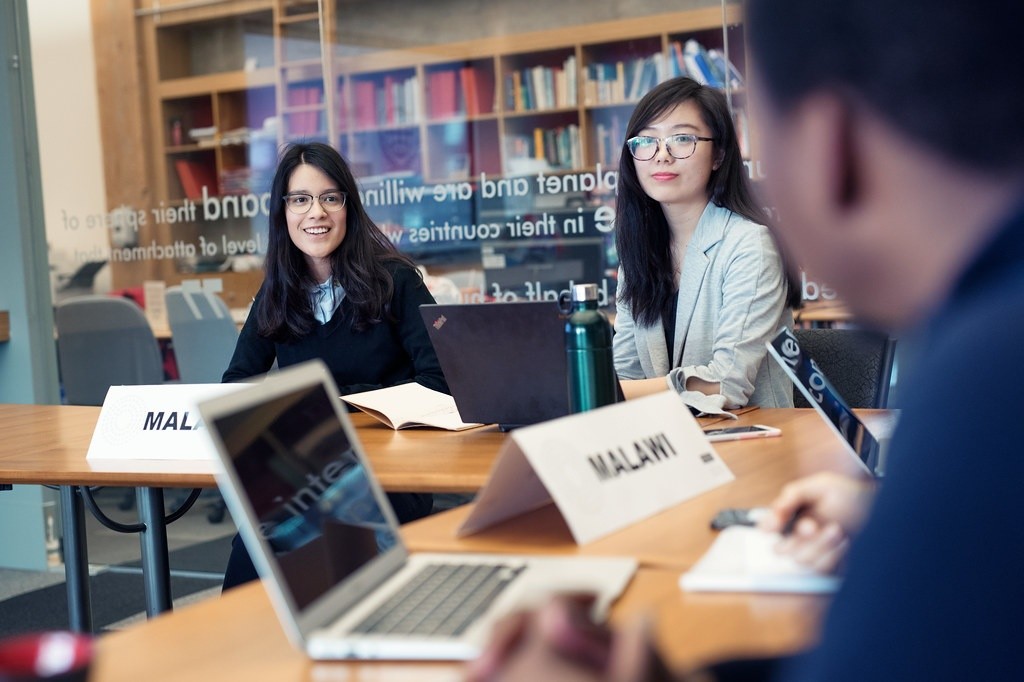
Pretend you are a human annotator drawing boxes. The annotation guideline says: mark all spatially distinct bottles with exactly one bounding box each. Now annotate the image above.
[565,284,615,415]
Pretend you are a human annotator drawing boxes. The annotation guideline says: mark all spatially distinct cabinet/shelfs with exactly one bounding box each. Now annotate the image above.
[91,0,858,339]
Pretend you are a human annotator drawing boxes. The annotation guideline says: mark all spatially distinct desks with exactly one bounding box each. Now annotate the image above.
[0,403,887,681]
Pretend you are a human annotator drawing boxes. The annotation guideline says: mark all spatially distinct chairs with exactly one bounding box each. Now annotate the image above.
[53,291,240,523]
[791,328,901,471]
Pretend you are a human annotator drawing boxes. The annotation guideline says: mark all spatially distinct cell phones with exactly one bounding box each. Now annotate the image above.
[702,425,781,443]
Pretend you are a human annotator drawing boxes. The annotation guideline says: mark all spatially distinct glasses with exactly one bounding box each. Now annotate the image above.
[626,135,715,161]
[283,191,349,214]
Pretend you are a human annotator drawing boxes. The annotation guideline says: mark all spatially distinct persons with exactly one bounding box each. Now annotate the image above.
[760,472,877,577]
[457,0,1024,682]
[221,141,451,593]
[611,78,806,408]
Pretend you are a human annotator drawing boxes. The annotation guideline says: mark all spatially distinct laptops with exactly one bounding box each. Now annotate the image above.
[194,359,639,661]
[419,300,626,433]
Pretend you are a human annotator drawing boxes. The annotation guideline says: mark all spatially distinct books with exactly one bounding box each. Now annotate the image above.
[339,382,484,432]
[169,43,857,329]
